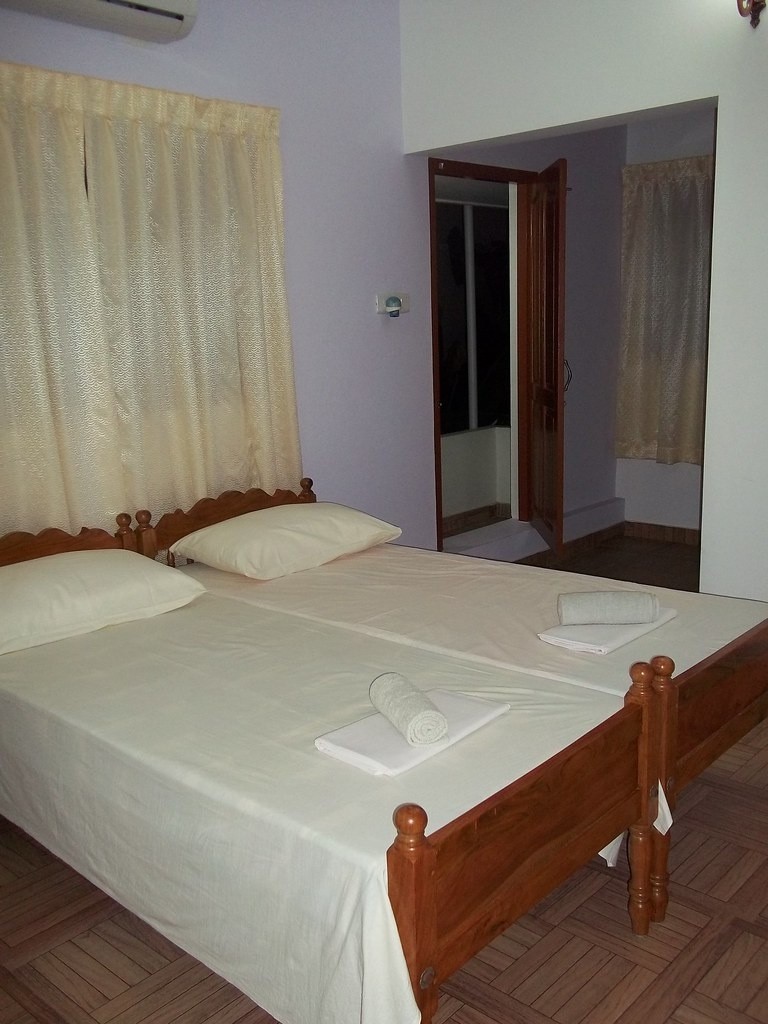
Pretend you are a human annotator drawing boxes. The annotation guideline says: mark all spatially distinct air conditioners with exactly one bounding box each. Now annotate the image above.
[0,0,200,44]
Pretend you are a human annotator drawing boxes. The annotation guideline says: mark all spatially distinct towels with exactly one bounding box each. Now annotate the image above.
[314,687,511,779]
[557,590,660,626]
[368,671,450,748]
[535,605,679,656]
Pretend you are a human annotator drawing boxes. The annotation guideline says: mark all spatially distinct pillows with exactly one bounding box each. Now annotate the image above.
[169,502,403,581]
[0,548,211,655]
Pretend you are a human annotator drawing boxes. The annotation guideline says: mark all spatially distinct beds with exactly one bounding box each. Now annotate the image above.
[0,474,768,1024]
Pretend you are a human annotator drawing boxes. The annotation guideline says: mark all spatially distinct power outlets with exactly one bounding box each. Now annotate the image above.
[375,292,410,315]
